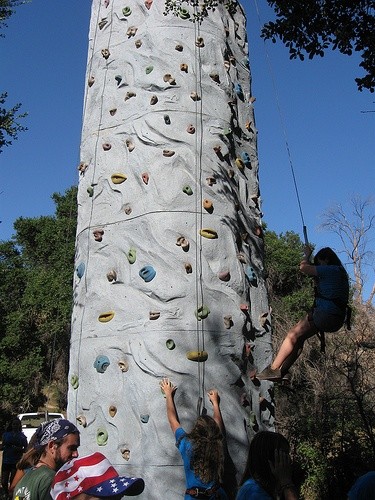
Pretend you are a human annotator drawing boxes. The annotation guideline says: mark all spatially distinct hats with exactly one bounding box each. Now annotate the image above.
[347,471,375,500]
[49,451,145,500]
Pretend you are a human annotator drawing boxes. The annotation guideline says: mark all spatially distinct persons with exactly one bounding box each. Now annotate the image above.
[235,430,298,500]
[159,378,229,500]
[0,416,145,500]
[347,471,375,500]
[255,243,349,381]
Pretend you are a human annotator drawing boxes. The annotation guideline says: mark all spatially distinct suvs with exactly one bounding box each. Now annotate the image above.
[16,410,66,450]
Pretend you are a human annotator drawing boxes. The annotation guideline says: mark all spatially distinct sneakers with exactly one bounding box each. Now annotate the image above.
[256,365,291,380]
